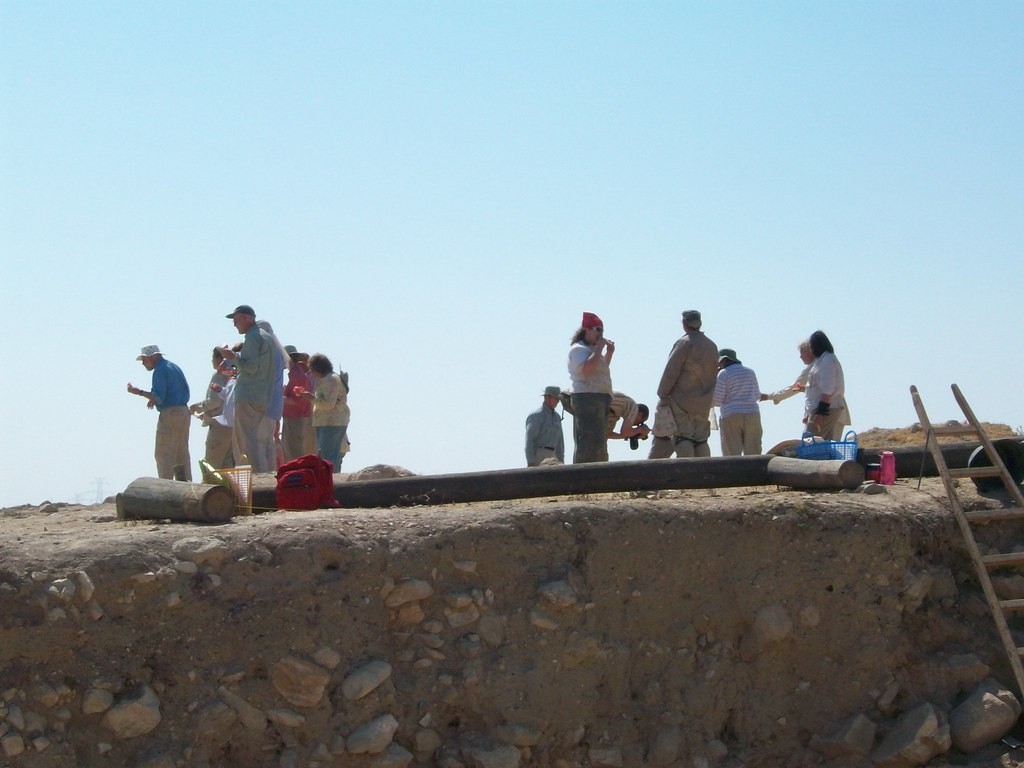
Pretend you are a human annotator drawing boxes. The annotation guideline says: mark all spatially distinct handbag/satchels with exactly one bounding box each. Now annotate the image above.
[652,402,679,437]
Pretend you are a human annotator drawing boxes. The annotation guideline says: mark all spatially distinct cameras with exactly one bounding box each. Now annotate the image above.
[629,435,648,450]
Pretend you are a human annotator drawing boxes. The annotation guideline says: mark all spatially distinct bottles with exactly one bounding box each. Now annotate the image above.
[878,450,896,485]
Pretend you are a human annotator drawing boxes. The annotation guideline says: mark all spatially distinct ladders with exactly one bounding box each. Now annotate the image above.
[910,382,1024,696]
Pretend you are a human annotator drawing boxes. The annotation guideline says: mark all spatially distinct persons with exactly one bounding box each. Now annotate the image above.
[567,312,615,464]
[561,388,651,462]
[711,348,763,456]
[190,305,350,475]
[759,330,852,442]
[657,310,719,458]
[526,387,564,467]
[127,345,193,482]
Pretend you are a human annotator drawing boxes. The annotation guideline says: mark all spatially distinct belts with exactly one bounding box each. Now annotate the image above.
[541,446,555,451]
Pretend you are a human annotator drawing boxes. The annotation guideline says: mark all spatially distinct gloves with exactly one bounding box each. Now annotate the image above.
[816,400,830,416]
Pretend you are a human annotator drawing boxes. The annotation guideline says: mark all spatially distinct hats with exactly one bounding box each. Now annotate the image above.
[541,386,562,399]
[340,366,349,393]
[718,348,741,364]
[283,346,309,368]
[226,305,255,319]
[136,345,165,360]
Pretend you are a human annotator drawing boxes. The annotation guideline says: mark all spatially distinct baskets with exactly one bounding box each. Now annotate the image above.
[199,453,252,516]
[795,430,858,462]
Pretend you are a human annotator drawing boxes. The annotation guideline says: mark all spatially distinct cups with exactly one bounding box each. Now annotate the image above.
[864,463,881,481]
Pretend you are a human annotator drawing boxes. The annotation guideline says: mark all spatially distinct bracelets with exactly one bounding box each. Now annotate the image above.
[140,390,144,396]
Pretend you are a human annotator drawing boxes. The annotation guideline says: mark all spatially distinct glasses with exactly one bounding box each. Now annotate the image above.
[589,327,603,332]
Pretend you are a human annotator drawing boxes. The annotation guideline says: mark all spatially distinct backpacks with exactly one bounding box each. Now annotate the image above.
[276,454,340,509]
